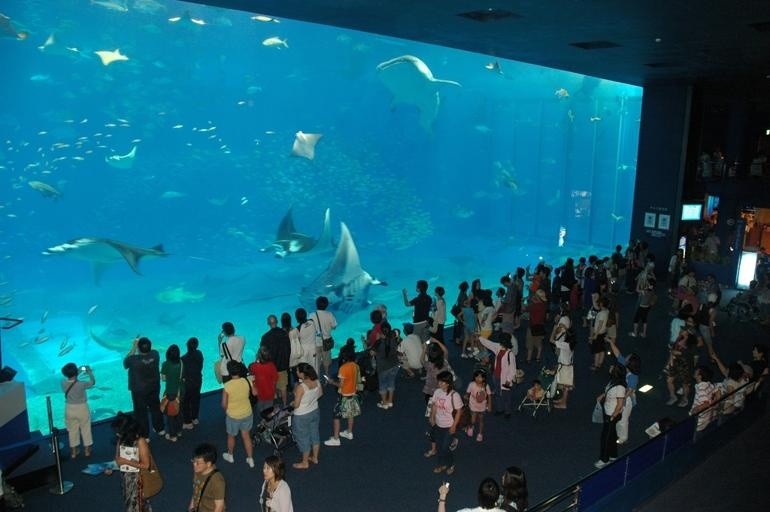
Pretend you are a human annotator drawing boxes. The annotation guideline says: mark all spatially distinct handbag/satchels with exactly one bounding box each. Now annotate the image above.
[323,336,334,350]
[250,394,257,405]
[451,305,461,318]
[592,401,603,423]
[160,395,180,416]
[453,408,468,428]
[141,457,162,498]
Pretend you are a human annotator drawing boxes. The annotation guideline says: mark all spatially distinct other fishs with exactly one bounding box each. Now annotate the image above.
[483,60,512,80]
[484,152,523,198]
[1,1,640,415]
[545,74,636,264]
[1,2,389,417]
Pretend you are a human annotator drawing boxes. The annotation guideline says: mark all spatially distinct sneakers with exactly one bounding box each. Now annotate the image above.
[477,435,483,442]
[377,401,393,409]
[596,458,617,467]
[629,332,646,338]
[468,429,474,437]
[71,448,92,459]
[666,397,689,408]
[309,457,318,463]
[461,347,479,358]
[324,438,340,446]
[183,419,198,430]
[293,464,309,468]
[526,359,539,365]
[223,452,234,463]
[245,458,254,468]
[425,451,437,457]
[434,465,455,474]
[340,431,353,439]
[554,399,566,408]
[154,428,181,442]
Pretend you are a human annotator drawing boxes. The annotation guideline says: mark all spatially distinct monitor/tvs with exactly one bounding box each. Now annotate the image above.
[682,203,703,221]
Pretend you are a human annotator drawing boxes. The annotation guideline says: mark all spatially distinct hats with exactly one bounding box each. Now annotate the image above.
[537,290,547,301]
[544,263,552,271]
[480,351,491,359]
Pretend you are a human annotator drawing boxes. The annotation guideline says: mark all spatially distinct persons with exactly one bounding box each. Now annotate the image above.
[104,411,153,511]
[61,363,96,458]
[494,466,528,512]
[160,344,183,442]
[123,337,166,443]
[180,337,203,430]
[258,456,294,512]
[187,443,227,512]
[216,300,404,473]
[437,478,507,512]
[399,207,769,474]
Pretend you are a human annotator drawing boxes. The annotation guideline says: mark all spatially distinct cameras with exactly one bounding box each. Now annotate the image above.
[425,336,432,344]
[219,330,225,338]
[508,274,512,278]
[81,366,86,371]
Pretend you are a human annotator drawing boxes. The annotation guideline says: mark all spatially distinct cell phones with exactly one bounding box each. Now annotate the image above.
[322,374,329,381]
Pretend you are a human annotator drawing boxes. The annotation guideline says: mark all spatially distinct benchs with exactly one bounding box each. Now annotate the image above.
[531,372,770,511]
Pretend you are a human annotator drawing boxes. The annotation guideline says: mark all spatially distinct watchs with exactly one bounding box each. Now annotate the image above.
[437,499,447,503]
